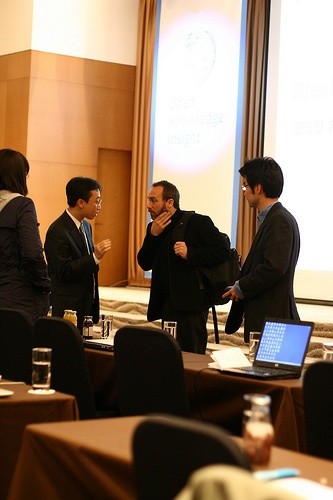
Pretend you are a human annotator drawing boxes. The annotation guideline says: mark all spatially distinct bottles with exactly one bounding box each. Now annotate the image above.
[82,315,93,339]
[242,394,275,468]
[63,309,78,328]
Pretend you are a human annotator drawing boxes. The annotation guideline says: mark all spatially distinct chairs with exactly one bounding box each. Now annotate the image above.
[0,308,333,500]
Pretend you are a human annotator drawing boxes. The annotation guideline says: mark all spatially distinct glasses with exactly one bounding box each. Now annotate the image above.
[240,184,251,192]
[88,196,102,206]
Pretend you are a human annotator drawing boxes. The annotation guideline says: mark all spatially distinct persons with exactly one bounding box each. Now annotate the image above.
[0,148,52,324]
[222,157,300,343]
[137,180,230,355]
[44,177,112,337]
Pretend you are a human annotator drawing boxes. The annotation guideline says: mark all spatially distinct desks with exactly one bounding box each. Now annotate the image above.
[5,416,333,500]
[85,345,308,448]
[0,378,78,500]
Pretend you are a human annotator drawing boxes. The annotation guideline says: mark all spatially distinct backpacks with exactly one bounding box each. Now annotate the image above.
[181,211,242,305]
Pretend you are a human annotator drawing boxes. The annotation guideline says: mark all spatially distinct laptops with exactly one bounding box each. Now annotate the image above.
[216,317,314,380]
[84,339,117,352]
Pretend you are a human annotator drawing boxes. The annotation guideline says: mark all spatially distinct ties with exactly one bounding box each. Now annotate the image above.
[80,223,96,298]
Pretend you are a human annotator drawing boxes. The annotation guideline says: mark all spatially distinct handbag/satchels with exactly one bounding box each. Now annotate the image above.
[225,286,243,335]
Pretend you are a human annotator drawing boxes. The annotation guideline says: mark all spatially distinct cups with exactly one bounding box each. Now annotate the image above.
[164,322,177,340]
[32,348,52,390]
[102,314,113,339]
[47,306,52,317]
[322,343,333,361]
[248,332,261,362]
